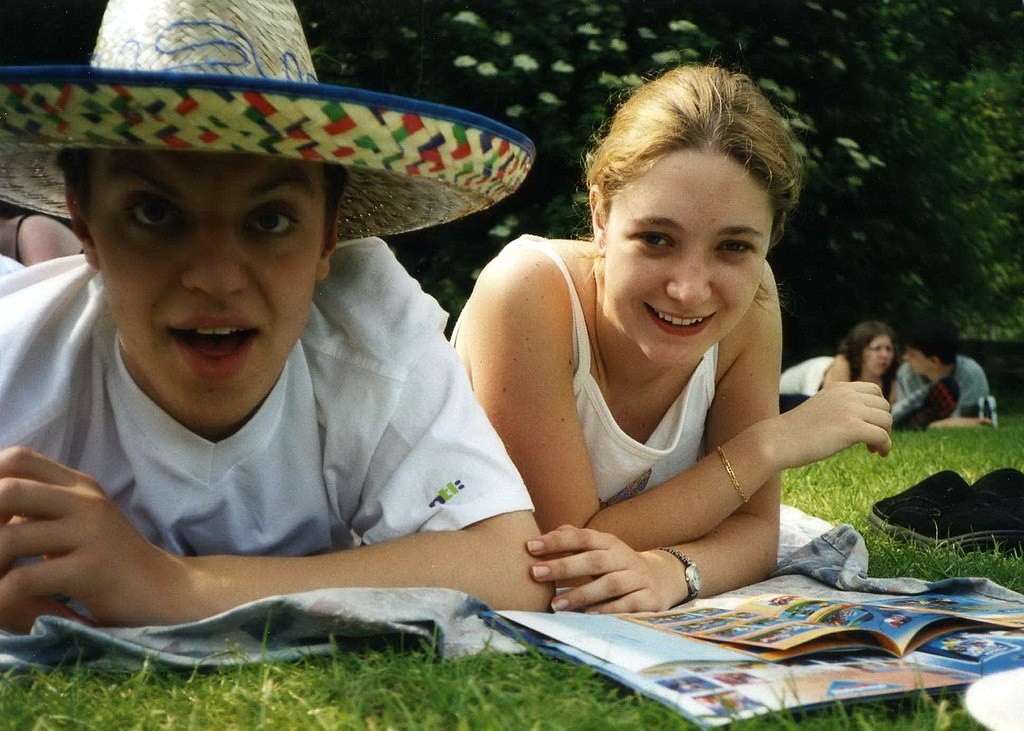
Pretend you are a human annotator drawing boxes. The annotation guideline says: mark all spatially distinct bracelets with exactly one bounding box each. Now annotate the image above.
[718,446,749,502]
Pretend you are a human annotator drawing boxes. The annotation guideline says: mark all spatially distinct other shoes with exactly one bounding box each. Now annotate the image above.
[869,468,1024,553]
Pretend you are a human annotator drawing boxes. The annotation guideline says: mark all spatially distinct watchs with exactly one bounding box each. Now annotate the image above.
[653,546,703,610]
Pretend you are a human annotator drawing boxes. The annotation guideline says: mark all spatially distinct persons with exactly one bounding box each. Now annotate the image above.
[452,64,896,615]
[0,140,558,636]
[779,310,992,428]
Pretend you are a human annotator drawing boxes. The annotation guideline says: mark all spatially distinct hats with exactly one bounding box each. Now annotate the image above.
[887,304,957,366]
[0,0,537,240]
